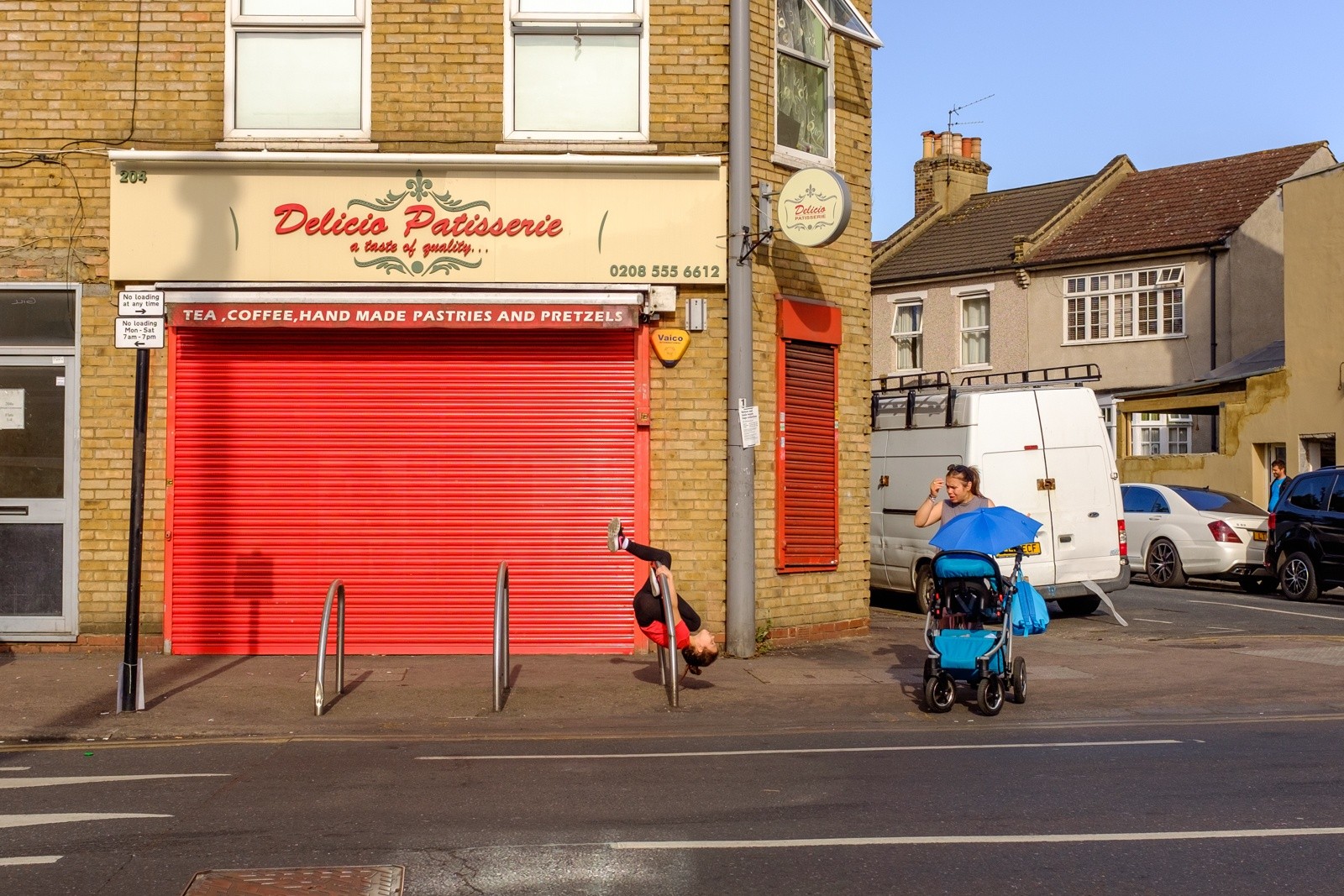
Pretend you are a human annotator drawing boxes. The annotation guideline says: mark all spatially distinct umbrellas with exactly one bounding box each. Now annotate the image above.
[928,506,1043,559]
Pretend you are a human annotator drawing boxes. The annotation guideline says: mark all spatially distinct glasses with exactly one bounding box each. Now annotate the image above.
[947,464,972,481]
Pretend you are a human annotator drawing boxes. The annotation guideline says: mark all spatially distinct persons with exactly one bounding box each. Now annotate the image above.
[915,465,996,632]
[1267,459,1293,512]
[608,517,718,682]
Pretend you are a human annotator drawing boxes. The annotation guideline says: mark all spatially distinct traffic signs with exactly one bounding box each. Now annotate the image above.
[114,317,165,349]
[118,292,165,317]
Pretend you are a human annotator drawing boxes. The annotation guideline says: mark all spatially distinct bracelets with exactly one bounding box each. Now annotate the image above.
[928,494,935,503]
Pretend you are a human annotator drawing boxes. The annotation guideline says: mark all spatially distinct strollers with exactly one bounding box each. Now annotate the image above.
[914,545,1029,715]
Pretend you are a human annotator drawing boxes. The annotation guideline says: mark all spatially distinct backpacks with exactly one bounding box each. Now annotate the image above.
[1003,569,1050,638]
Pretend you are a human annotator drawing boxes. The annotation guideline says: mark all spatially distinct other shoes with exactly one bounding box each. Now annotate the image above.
[650,566,661,596]
[607,518,625,552]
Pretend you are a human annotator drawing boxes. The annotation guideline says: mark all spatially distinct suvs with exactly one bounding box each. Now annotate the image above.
[1263,466,1344,603]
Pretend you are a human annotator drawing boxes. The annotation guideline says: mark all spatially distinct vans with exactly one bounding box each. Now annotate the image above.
[869,363,1132,621]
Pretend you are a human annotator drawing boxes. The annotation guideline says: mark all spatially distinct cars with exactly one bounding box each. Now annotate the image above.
[1115,482,1280,593]
